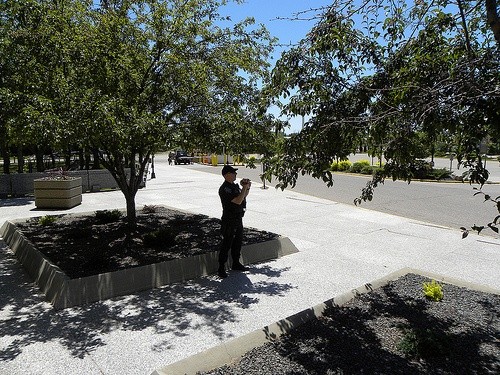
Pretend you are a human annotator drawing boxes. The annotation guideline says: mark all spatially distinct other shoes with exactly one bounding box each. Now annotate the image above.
[218,265,227,278]
[232,262,249,271]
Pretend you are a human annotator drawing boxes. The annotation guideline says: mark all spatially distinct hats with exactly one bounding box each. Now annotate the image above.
[222,166,238,175]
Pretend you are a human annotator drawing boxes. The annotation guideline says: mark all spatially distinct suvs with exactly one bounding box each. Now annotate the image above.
[174,150,191,165]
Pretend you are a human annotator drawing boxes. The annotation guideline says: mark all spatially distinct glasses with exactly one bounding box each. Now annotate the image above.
[230,171,235,174]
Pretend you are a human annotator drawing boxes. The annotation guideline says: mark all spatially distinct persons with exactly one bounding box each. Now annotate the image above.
[219,165,251,278]
[168,151,194,166]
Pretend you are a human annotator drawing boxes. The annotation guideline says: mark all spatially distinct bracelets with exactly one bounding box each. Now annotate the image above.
[246,188,250,190]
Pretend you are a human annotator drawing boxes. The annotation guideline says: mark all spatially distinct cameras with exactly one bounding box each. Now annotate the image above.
[242,178,250,190]
